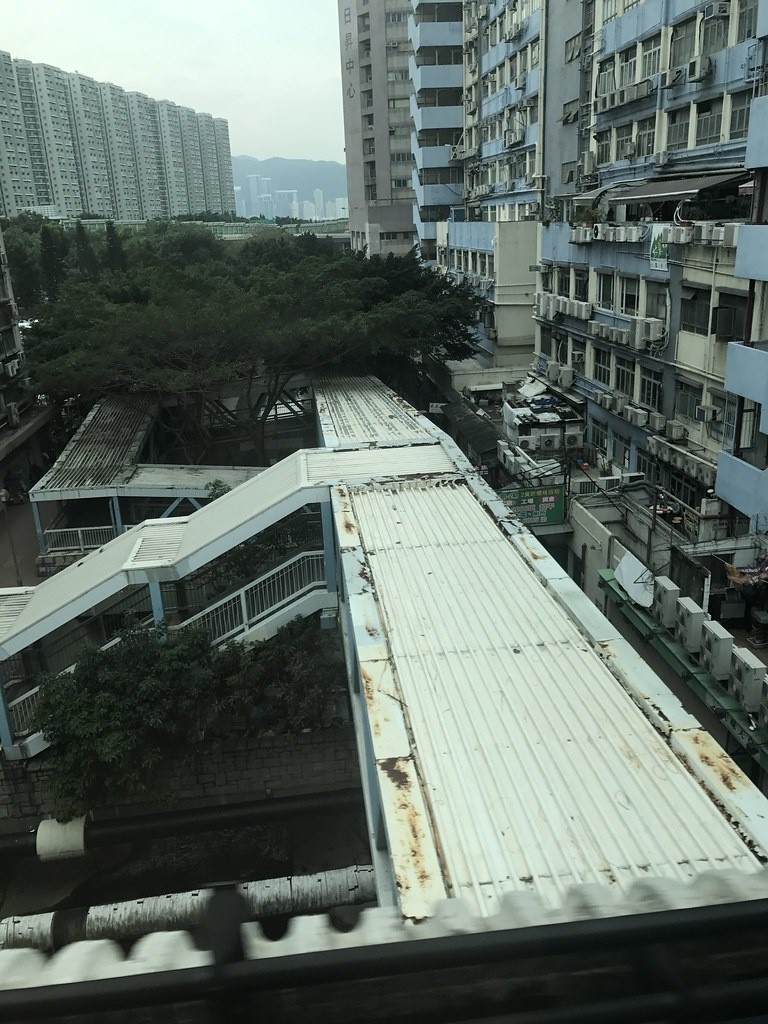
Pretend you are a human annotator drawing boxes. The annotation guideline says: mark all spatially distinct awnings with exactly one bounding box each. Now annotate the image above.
[607,168,763,207]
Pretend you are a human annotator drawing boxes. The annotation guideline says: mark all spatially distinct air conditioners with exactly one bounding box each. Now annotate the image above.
[450,63,490,199]
[497,434,560,488]
[22,378,32,390]
[661,69,678,88]
[564,431,584,447]
[580,151,593,176]
[530,203,537,212]
[652,576,681,628]
[386,41,397,48]
[8,303,19,318]
[656,151,668,165]
[508,76,534,145]
[593,80,653,115]
[570,222,745,247]
[6,402,19,427]
[598,476,621,491]
[705,2,729,20]
[623,142,634,156]
[535,292,662,349]
[546,351,712,439]
[675,596,704,652]
[525,173,533,185]
[728,648,766,712]
[699,621,735,680]
[478,5,486,19]
[571,478,595,494]
[505,22,524,43]
[647,437,715,486]
[686,54,709,83]
[621,472,646,486]
[4,351,24,377]
